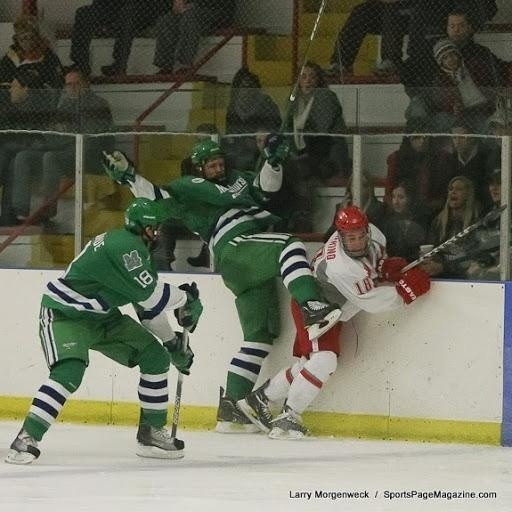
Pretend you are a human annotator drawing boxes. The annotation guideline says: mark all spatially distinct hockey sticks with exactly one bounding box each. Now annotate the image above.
[400,205,506,273]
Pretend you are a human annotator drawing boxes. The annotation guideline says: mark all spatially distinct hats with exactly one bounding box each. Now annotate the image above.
[485,108,512,127]
[434,40,461,68]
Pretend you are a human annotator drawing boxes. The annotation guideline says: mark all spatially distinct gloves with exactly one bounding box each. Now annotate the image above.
[167,331,194,374]
[396,269,430,303]
[381,258,408,282]
[177,283,203,332]
[100,150,136,185]
[261,140,290,166]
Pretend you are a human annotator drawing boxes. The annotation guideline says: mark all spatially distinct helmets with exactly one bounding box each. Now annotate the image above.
[124,199,170,251]
[336,207,372,256]
[190,142,228,183]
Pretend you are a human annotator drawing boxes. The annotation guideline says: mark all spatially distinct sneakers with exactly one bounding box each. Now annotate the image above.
[187,244,210,267]
[137,423,183,450]
[369,59,400,76]
[10,431,40,459]
[216,381,311,438]
[302,301,339,328]
[320,64,353,77]
[100,64,127,77]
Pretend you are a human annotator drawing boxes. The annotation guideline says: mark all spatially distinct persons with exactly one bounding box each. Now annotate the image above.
[0,0,510,283]
[9,197,203,458]
[235,205,431,436]
[100,137,341,428]
